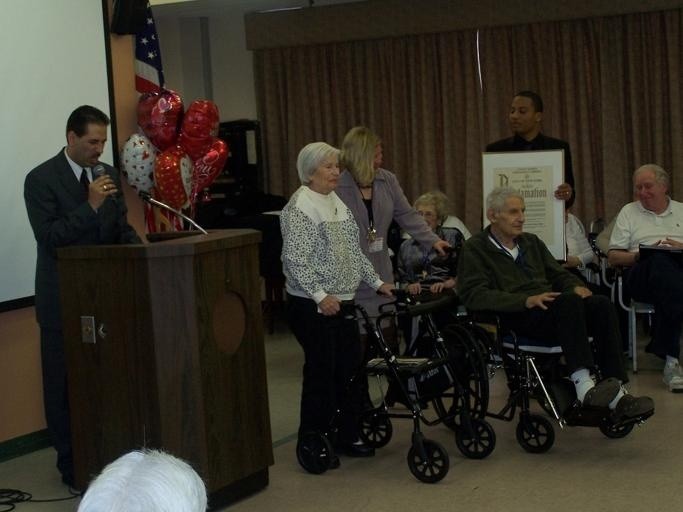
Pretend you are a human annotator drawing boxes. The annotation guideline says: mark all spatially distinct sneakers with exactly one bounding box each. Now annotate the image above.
[584,377,620,407]
[616,394,655,417]
[662,363,683,392]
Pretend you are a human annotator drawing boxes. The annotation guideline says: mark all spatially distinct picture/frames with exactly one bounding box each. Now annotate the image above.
[480,149,567,263]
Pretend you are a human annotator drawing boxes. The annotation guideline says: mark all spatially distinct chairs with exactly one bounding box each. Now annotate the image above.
[586,215,656,374]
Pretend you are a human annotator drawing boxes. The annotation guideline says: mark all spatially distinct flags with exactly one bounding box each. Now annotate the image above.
[133,3,165,97]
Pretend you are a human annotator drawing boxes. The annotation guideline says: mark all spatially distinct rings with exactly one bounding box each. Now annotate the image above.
[566,192,569,195]
[102,184,108,190]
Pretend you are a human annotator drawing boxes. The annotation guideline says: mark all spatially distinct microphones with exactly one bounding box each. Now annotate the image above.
[92,164,120,206]
[138,189,209,235]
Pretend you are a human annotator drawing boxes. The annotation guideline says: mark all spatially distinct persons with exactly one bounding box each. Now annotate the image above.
[335,126,450,356]
[455,186,654,418]
[397,189,466,295]
[484,89,576,211]
[606,163,683,393]
[278,141,396,469]
[557,209,596,282]
[75,445,209,511]
[23,104,144,486]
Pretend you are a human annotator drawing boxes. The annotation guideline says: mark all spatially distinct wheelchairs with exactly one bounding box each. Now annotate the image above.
[295,246,655,485]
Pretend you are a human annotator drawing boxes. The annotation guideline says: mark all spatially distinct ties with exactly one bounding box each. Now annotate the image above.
[80,168,90,199]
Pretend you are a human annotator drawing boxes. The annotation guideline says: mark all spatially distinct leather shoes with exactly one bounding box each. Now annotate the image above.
[330,434,374,457]
[294,440,341,469]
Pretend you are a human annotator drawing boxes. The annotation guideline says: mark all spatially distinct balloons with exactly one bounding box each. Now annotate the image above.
[119,87,229,210]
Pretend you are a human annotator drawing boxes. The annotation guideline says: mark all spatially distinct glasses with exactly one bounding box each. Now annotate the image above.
[418,211,438,218]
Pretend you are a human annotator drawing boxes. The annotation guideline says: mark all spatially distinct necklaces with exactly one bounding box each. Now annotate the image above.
[358,186,377,242]
